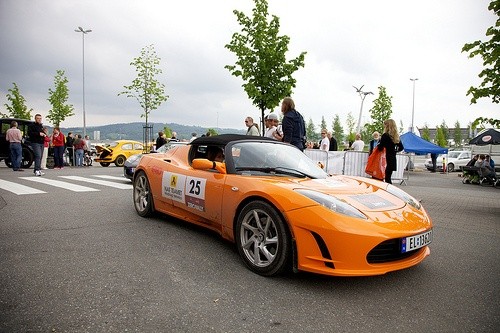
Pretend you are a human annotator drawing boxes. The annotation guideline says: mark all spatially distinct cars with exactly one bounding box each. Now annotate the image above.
[123,141,207,182]
[93,138,154,167]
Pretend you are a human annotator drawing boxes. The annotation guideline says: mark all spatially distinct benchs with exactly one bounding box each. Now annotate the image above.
[459,166,494,187]
[391,177,409,186]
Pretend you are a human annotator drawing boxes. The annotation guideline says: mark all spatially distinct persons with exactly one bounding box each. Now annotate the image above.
[156,132,167,150]
[313,142,319,149]
[41,127,51,169]
[327,131,337,151]
[467,154,494,185]
[171,132,180,142]
[372,119,404,184]
[264,114,283,140]
[245,117,259,136]
[53,128,65,170]
[6,121,24,171]
[319,129,330,151]
[28,114,47,176]
[281,98,306,152]
[350,133,364,151]
[67,132,92,167]
[206,132,211,136]
[368,131,381,156]
[208,147,223,162]
[432,153,436,168]
[190,133,197,142]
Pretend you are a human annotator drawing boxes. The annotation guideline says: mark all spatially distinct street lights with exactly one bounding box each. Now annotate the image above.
[74,26,94,139]
[410,78,419,133]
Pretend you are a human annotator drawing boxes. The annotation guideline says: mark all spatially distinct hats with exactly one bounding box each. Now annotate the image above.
[268,114,277,120]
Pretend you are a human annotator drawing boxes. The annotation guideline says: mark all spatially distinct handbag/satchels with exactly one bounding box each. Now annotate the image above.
[364,146,387,180]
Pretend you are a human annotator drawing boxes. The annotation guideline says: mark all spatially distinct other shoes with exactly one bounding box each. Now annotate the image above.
[53,166,63,170]
[35,170,45,176]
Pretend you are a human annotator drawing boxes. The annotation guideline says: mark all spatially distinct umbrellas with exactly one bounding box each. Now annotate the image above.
[468,128,500,163]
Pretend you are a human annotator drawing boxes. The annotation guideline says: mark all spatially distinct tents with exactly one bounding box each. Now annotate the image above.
[399,131,448,173]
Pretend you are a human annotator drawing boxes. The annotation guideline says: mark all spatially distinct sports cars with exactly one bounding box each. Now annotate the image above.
[132,133,432,278]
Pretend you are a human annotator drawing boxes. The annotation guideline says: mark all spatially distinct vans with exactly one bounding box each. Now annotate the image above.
[0,118,35,169]
[425,150,471,172]
